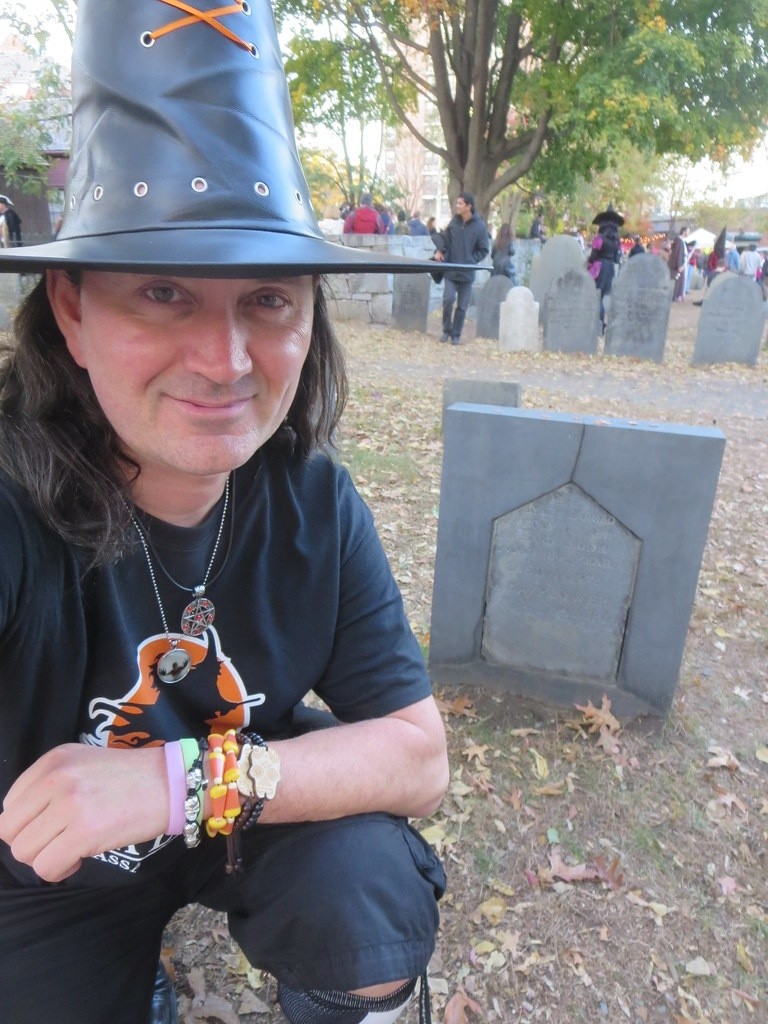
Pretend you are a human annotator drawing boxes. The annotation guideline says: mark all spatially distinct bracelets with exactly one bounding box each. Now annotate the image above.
[165,729,281,874]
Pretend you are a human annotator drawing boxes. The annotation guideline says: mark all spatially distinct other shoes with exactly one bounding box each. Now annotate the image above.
[440,332,449,342]
[451,335,460,344]
[153,957,177,1024]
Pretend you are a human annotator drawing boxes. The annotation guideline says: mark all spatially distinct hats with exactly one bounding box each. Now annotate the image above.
[592,201,624,227]
[0,1,496,280]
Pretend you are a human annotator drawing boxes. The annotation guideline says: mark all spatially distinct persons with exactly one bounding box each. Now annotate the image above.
[628,235,645,257]
[0,195,26,248]
[530,213,547,242]
[431,192,489,343]
[667,225,768,306]
[588,201,625,334]
[490,224,517,287]
[1,0,496,1024]
[337,195,437,235]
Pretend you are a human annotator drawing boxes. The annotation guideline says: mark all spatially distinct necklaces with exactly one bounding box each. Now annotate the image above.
[122,473,236,684]
